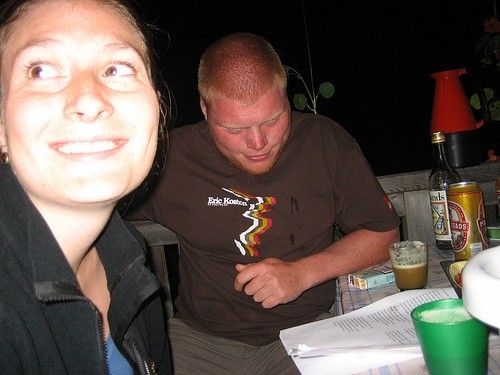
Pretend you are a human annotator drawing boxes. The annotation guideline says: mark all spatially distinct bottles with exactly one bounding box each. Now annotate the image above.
[428,132,463,250]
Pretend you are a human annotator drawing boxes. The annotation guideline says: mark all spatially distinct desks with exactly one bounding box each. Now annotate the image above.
[334,245,500,375]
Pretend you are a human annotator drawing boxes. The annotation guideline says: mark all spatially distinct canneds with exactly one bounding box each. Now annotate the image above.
[446,181,489,262]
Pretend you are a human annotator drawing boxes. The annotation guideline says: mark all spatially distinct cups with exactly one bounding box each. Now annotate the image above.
[411,299,489,375]
[388,239,427,292]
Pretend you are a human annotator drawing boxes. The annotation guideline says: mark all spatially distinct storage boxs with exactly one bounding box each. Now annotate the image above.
[347,265,395,290]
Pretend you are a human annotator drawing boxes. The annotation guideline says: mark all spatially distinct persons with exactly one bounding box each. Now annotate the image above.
[0,1,176,375]
[118,32,400,375]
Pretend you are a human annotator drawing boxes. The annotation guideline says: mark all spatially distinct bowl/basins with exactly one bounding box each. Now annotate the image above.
[461,246,500,329]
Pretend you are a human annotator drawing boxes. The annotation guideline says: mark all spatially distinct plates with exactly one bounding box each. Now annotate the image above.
[449,260,467,287]
